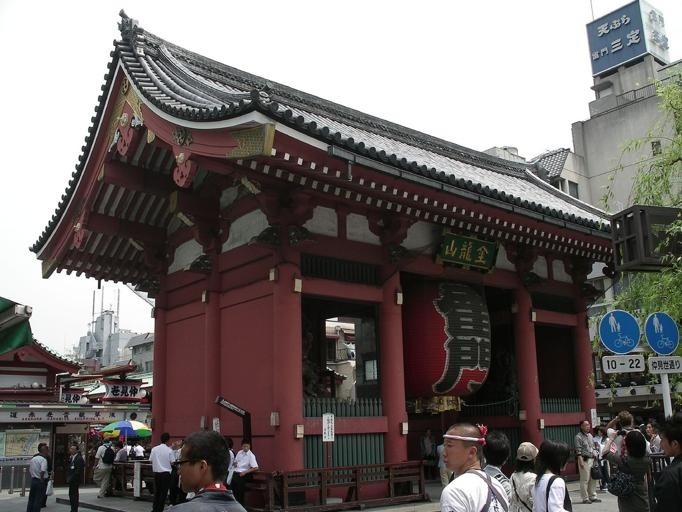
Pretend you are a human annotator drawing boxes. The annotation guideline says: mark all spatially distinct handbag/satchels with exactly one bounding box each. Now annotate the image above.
[607,472,635,496]
[591,464,604,479]
[45,479,54,495]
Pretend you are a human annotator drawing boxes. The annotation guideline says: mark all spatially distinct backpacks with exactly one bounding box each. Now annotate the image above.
[101,443,115,464]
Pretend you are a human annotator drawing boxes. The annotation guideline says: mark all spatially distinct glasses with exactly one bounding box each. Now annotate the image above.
[171,459,192,469]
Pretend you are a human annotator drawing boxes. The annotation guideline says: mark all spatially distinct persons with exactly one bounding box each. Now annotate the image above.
[419,427,437,479]
[531,439,572,511]
[439,421,510,511]
[510,440,539,511]
[436,442,453,489]
[573,409,682,511]
[27,430,260,512]
[482,428,511,506]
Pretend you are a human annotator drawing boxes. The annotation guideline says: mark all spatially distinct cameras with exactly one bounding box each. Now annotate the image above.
[617,431,626,435]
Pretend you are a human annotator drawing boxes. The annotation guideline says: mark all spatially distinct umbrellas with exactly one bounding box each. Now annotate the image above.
[98,419,153,446]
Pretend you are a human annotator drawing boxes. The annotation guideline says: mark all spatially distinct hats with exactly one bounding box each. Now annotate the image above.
[517,442,539,461]
